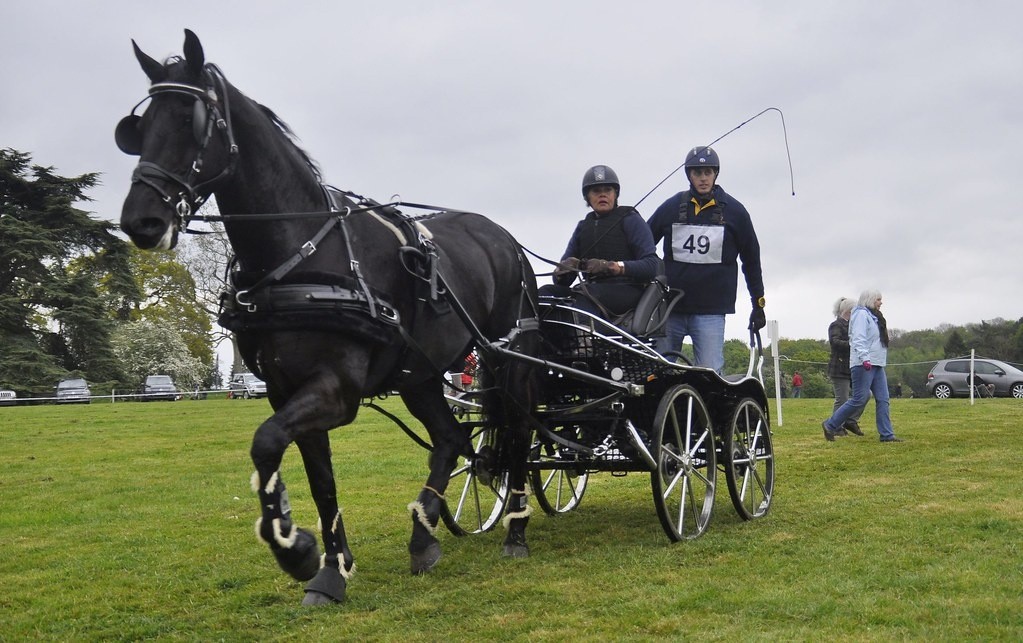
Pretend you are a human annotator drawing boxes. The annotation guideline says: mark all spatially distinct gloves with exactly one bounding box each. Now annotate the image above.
[586,258,615,276]
[863,361,871,370]
[555,257,581,281]
[747,297,766,333]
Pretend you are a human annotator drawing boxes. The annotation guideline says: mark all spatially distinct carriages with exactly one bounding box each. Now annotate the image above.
[113,28,779,611]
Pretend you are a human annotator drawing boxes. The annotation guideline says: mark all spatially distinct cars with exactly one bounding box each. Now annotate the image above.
[926,358,1023,399]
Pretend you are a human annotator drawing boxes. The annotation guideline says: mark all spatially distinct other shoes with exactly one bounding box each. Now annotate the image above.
[835,427,849,437]
[822,422,836,442]
[880,438,902,443]
[842,422,864,436]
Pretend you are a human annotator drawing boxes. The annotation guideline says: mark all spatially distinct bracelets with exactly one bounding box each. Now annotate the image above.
[751,297,765,308]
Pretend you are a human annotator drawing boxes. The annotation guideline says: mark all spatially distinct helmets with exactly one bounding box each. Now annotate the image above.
[582,166,620,196]
[685,146,720,178]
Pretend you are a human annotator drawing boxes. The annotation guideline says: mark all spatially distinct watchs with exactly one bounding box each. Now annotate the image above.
[618,261,625,275]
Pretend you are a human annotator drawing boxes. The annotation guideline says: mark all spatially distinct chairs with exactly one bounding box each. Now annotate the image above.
[603,258,685,338]
[965,378,989,398]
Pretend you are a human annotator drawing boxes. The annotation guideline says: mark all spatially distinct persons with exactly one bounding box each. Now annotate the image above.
[780,371,788,398]
[538,166,659,357]
[967,369,995,398]
[822,287,905,442]
[176,386,235,400]
[647,146,766,375]
[826,296,864,437]
[791,371,802,398]
[446,352,476,413]
[896,383,903,397]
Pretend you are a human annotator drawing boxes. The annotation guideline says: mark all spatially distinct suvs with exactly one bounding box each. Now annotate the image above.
[137,374,177,402]
[229,372,267,400]
[52,377,92,404]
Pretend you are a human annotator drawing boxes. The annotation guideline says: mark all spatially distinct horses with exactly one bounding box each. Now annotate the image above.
[120,28,536,608]
